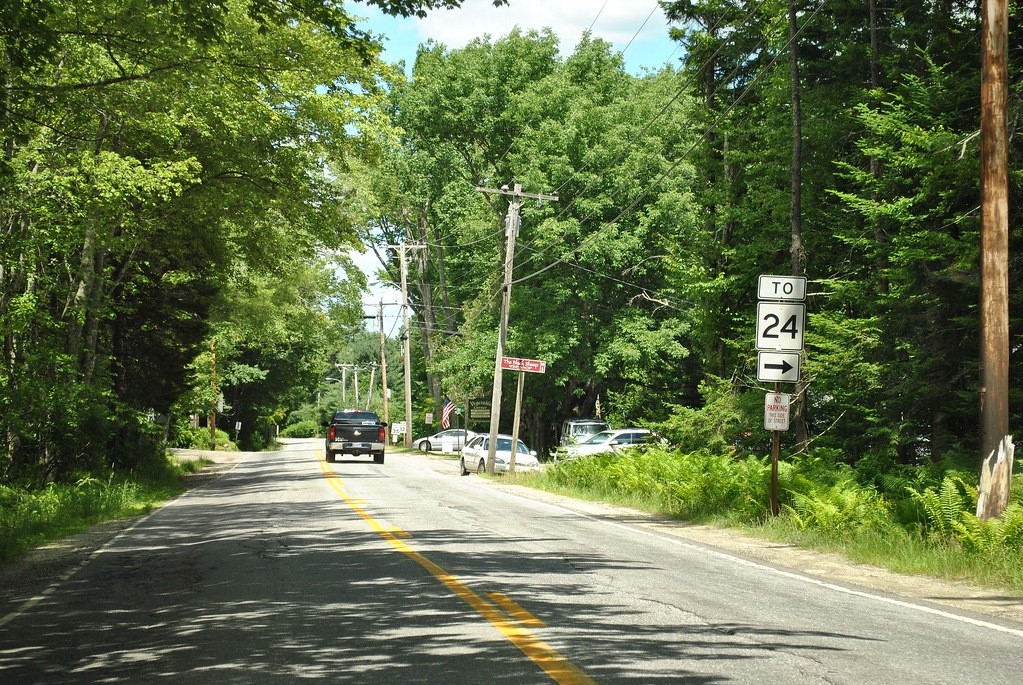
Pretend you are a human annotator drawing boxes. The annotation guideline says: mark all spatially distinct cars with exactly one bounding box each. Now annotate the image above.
[412,429,478,452]
[554,419,677,467]
[460,433,542,476]
[321,411,388,464]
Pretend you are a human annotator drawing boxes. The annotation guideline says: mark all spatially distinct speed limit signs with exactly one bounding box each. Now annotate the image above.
[755,302,807,353]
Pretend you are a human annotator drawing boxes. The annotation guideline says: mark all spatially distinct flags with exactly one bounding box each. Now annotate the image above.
[442,397,456,430]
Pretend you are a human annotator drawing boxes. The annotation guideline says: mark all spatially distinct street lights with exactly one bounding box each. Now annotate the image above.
[369,241,427,448]
[325,360,359,412]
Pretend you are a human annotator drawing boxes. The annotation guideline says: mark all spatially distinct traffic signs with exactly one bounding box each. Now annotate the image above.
[764,392,790,431]
